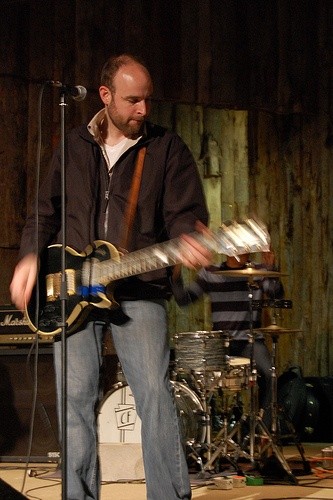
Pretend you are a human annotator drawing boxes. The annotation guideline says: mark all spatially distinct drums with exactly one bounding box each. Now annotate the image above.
[172,330,227,374]
[210,356,254,392]
[95,380,207,470]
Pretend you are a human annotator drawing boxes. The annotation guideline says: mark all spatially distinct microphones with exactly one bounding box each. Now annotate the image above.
[46,80,87,102]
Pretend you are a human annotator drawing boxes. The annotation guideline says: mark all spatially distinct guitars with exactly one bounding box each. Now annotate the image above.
[23,216,272,343]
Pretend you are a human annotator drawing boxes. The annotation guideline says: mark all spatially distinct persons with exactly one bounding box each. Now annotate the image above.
[8,51,216,500]
[169,218,288,479]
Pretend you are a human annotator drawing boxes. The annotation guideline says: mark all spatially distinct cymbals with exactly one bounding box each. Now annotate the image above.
[245,314,303,334]
[210,263,290,278]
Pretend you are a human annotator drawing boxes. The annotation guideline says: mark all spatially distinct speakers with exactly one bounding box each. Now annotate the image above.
[0,347,61,464]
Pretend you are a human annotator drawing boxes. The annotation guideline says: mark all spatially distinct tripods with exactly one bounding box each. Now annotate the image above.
[186,276,313,486]
